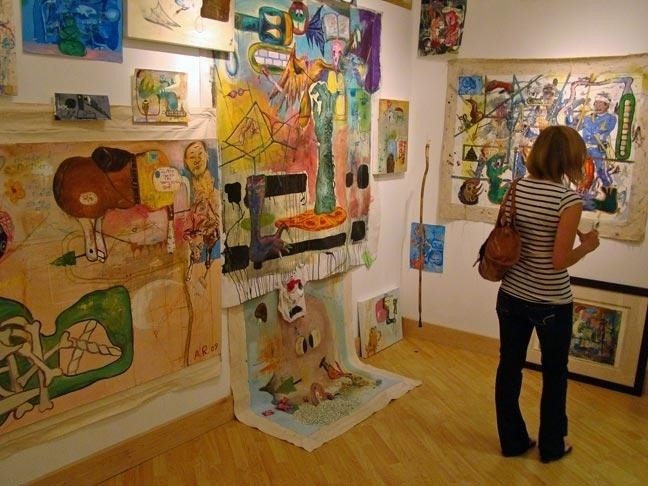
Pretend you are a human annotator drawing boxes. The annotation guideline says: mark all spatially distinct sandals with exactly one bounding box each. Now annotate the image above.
[527,436,537,451]
[562,443,573,456]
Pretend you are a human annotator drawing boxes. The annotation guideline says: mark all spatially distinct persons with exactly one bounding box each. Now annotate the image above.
[493,123,602,462]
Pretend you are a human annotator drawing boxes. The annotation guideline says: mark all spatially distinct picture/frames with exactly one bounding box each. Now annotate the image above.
[522,276,648,397]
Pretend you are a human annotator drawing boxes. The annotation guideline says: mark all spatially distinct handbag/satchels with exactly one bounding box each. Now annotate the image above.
[477,176,525,282]
